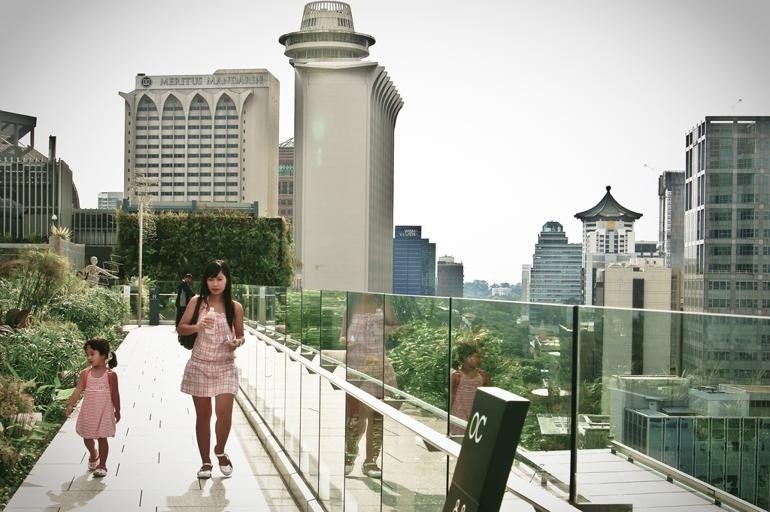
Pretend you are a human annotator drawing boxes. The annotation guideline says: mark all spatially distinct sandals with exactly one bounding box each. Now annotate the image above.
[88,449,99,470]
[93,463,108,476]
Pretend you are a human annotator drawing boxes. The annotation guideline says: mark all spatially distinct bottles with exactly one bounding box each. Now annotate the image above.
[204,306,216,335]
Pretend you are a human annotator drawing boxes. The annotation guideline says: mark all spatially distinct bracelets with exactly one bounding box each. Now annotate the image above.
[238,337,243,347]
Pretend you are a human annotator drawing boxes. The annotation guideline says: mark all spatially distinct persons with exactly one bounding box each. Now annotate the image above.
[66,337,121,477]
[339,293,401,479]
[177,259,245,478]
[84,256,119,288]
[448,341,494,434]
[175,273,198,329]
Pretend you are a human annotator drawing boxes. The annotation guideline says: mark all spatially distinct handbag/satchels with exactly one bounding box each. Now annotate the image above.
[177,312,200,349]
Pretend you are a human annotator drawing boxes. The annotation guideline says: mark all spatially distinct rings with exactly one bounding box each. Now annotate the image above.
[204,322,207,326]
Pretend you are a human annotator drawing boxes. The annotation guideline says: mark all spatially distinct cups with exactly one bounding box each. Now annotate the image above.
[226,334,237,347]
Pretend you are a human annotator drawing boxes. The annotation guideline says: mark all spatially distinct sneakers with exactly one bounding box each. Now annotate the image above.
[345,446,358,475]
[197,462,213,479]
[214,446,232,475]
[361,460,382,478]
